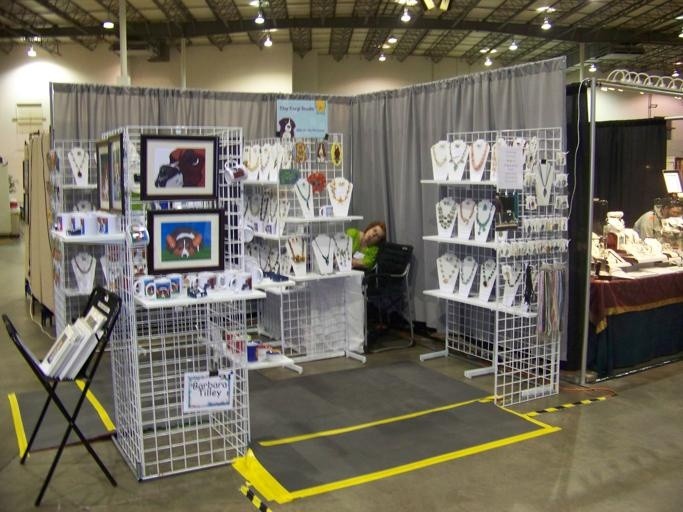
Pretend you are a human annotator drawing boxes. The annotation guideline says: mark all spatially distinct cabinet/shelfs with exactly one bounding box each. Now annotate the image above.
[283,214,364,286]
[417,175,540,326]
[137,286,294,376]
[51,184,126,298]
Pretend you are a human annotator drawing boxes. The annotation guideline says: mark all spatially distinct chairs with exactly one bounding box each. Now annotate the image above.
[360,241,419,356]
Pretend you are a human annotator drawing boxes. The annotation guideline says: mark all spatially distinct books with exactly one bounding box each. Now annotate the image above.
[40,304,108,380]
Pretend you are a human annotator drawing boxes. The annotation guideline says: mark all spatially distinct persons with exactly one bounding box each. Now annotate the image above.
[633,200,683,241]
[345,222,385,267]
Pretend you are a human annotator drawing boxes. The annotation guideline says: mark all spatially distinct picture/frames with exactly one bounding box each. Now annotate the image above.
[94,133,124,213]
[139,135,225,278]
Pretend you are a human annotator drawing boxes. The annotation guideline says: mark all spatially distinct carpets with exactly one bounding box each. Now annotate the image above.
[10,353,115,466]
[228,355,560,505]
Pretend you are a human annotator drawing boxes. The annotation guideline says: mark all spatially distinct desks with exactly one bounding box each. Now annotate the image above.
[592,265,682,378]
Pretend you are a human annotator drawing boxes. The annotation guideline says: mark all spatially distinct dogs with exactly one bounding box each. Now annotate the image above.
[164,226,205,259]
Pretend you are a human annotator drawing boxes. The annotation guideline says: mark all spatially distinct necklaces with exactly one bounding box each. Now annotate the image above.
[432,142,551,288]
[296,183,314,210]
[334,236,351,264]
[315,239,332,265]
[330,178,351,201]
[243,147,288,270]
[74,255,94,273]
[70,151,86,177]
[289,240,306,262]
